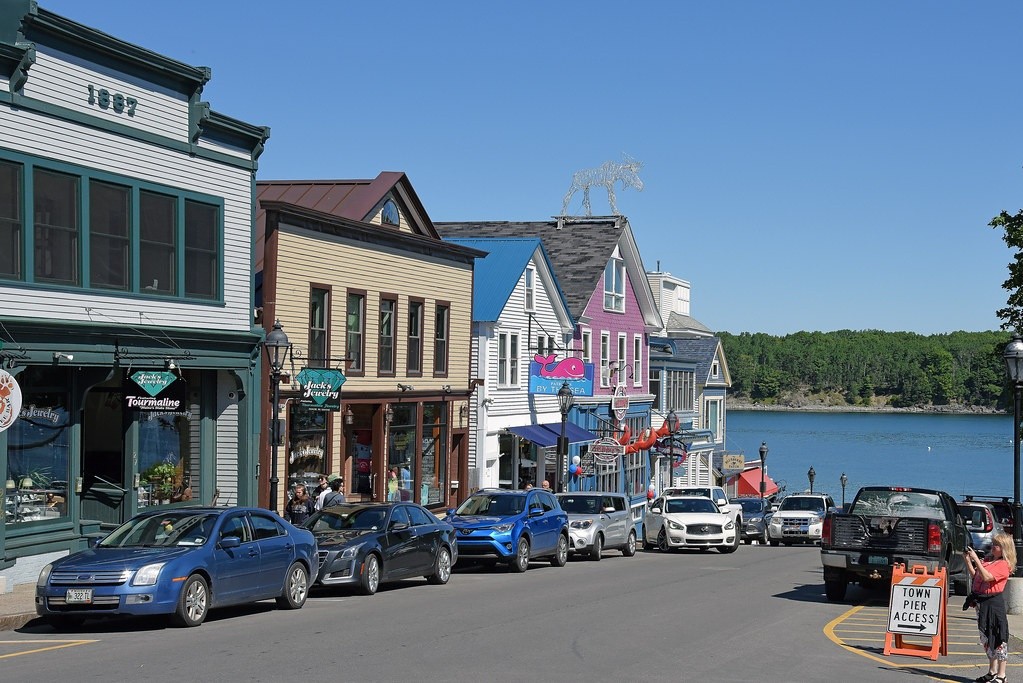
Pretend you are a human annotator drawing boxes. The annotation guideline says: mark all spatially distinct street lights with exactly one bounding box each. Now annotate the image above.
[1001,331,1023,578]
[840,471,848,509]
[758,441,769,499]
[556,380,574,493]
[262,318,291,512]
[666,409,677,488]
[807,466,817,494]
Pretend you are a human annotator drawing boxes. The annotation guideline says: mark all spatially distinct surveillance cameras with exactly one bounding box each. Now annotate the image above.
[228,392,235,399]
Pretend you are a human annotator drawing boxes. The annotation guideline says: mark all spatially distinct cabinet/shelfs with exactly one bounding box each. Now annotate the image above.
[5,491,47,525]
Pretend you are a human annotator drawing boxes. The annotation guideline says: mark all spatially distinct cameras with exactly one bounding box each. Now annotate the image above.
[963,547,971,553]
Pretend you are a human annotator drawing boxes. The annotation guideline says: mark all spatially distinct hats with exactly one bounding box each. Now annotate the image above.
[327,474,342,482]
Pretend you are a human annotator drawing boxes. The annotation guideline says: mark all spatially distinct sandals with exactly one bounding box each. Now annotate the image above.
[986,676,1008,683]
[975,673,998,683]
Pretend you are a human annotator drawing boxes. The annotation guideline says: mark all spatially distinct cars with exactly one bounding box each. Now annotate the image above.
[553,492,637,561]
[35,506,320,630]
[641,495,740,554]
[291,501,459,595]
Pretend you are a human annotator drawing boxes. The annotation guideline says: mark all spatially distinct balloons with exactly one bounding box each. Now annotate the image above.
[648,485,656,499]
[570,456,582,475]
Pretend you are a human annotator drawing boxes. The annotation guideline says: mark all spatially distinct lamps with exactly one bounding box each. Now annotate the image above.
[398,382,415,392]
[614,363,634,379]
[54,352,73,361]
[165,358,175,369]
[344,404,354,424]
[460,401,469,417]
[442,385,451,393]
[386,404,394,422]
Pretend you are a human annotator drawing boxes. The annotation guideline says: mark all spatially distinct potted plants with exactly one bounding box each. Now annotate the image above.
[139,463,177,498]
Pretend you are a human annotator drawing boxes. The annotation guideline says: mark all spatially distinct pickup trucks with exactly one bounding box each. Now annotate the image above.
[659,484,743,535]
[819,486,974,602]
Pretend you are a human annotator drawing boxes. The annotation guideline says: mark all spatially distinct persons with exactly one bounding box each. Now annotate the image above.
[963,534,1017,683]
[312,473,345,512]
[525,484,532,490]
[541,480,556,494]
[283,484,315,526]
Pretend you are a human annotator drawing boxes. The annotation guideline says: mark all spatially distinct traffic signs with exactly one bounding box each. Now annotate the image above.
[885,583,941,636]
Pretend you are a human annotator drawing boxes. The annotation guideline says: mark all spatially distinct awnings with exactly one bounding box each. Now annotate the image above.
[738,469,778,497]
[502,421,601,450]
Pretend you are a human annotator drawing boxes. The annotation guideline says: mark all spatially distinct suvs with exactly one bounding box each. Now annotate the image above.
[440,488,570,573]
[956,493,1015,560]
[729,498,773,546]
[768,492,835,547]
